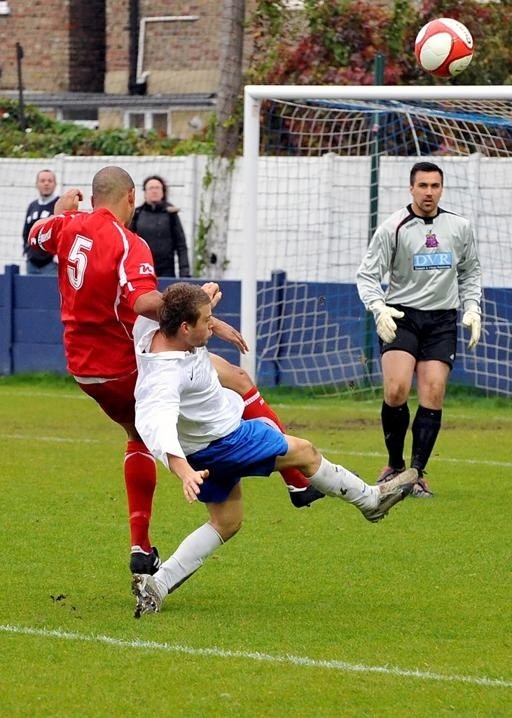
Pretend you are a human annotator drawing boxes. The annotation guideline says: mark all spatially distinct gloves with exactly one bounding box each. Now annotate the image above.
[462,308,483,350]
[370,305,404,343]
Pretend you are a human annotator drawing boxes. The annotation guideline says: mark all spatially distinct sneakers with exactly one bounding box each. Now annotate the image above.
[377,466,405,486]
[130,545,163,573]
[128,575,164,624]
[413,477,431,496]
[364,470,421,526]
[288,485,325,508]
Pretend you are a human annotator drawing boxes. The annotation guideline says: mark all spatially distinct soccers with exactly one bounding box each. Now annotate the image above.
[413,18,473,81]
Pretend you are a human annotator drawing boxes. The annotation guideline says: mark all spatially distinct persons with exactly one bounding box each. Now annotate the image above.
[27,167,359,575]
[129,177,189,278]
[23,171,61,274]
[131,281,418,620]
[356,162,483,499]
